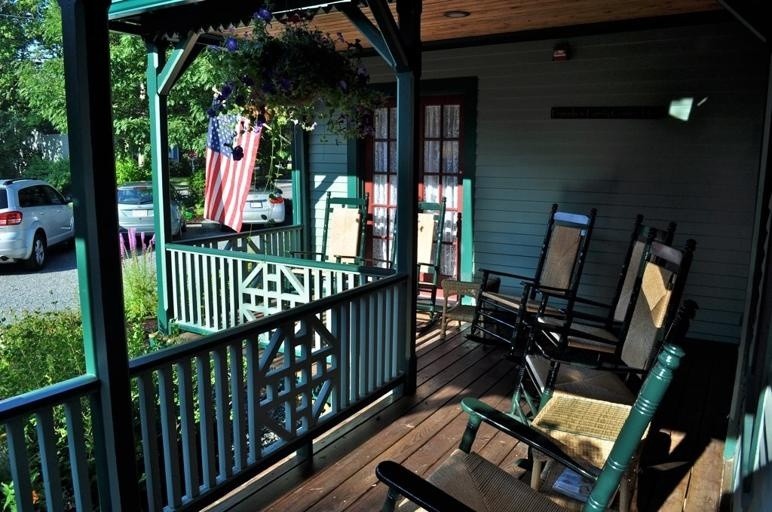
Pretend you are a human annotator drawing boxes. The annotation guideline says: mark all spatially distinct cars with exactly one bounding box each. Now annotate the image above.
[172,184,199,208]
[240,176,287,228]
[116,181,187,242]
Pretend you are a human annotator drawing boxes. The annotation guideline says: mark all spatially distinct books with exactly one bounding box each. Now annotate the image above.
[551,467,620,508]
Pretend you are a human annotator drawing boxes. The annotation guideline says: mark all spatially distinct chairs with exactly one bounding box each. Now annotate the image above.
[361,198,446,332]
[465,202,597,364]
[512,214,678,365]
[285,192,369,309]
[509,228,696,429]
[374,298,701,512]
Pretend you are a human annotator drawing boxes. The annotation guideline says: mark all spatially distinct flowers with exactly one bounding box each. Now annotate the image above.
[119,228,158,317]
[199,9,391,199]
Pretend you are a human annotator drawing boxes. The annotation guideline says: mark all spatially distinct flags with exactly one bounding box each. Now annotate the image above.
[204,93,263,233]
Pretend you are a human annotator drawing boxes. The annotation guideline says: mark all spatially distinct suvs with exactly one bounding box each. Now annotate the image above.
[0,177,75,271]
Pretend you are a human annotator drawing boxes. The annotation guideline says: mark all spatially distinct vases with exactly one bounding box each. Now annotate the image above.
[260,79,317,106]
[129,318,157,334]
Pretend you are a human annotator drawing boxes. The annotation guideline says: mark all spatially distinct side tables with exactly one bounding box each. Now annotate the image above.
[440,276,501,339]
[529,391,651,512]
